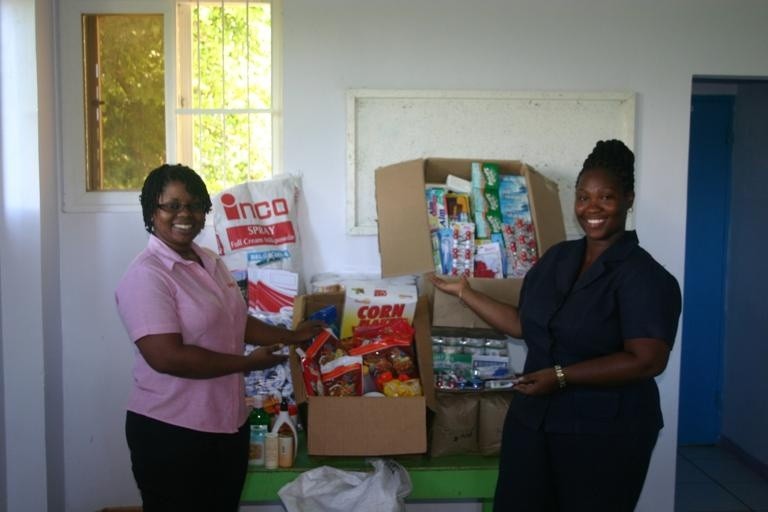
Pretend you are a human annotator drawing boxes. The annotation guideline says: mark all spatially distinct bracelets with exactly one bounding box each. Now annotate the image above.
[459,286,472,308]
[555,366,567,389]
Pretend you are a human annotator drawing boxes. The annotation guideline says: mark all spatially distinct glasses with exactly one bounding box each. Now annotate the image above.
[156,202,206,213]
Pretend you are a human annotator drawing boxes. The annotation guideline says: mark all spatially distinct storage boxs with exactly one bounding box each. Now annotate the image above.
[288,289,438,460]
[374,154,567,329]
[429,371,524,455]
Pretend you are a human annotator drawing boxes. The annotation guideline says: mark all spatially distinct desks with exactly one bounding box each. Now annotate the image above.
[241,429,504,512]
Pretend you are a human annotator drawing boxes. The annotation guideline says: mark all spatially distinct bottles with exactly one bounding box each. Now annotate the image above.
[247,393,299,470]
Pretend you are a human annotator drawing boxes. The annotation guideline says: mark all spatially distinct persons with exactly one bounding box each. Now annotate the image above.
[429,139,682,512]
[115,164,329,512]
